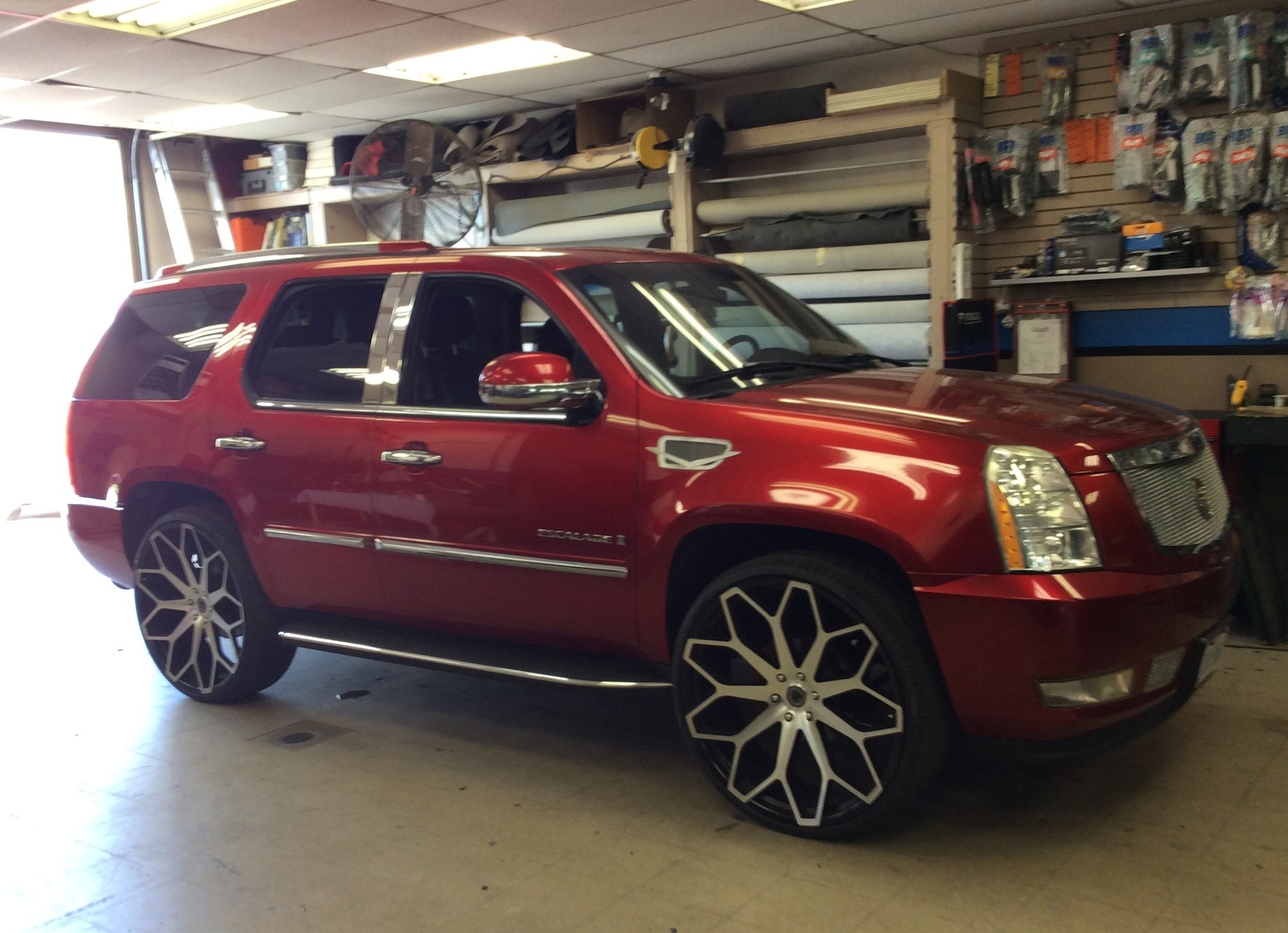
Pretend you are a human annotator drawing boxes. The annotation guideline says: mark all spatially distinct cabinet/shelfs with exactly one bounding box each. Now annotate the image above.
[1225,411,1288,650]
[218,99,982,369]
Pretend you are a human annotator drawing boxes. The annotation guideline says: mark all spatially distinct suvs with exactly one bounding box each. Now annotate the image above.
[63,239,1238,840]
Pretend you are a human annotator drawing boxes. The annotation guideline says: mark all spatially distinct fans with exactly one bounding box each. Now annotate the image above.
[348,118,483,247]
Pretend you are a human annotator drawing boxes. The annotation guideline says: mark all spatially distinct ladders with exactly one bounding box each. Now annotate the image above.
[147,139,234,264]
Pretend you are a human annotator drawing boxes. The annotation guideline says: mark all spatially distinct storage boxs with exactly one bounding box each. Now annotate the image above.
[1053,221,1167,275]
[239,136,349,194]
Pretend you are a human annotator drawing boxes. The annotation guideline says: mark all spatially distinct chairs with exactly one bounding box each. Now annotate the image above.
[540,314,595,379]
